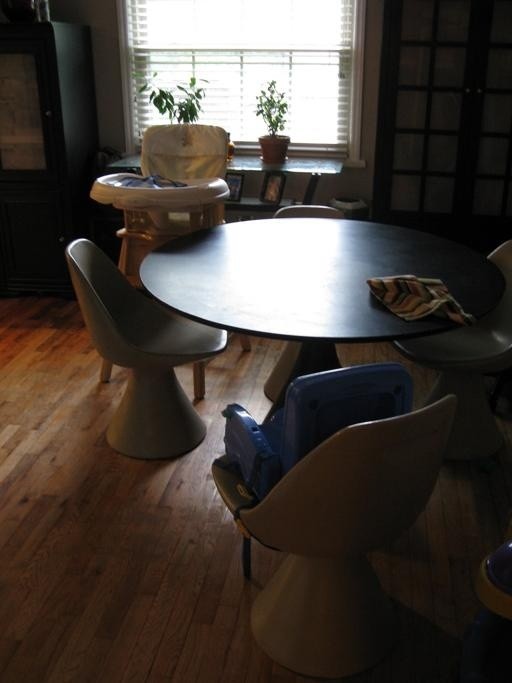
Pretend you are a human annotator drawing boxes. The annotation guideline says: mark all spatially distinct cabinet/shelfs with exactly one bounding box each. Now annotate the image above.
[0,22,98,295]
[368,1,511,260]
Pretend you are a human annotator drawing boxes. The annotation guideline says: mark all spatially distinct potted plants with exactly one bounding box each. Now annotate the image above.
[255,80,291,161]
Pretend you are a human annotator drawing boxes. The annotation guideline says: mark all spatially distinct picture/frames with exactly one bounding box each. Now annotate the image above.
[224,173,244,203]
[259,170,286,205]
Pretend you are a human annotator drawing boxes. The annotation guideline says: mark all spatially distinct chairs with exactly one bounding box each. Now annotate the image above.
[392,237,511,464]
[90,123,250,399]
[271,205,344,219]
[209,363,457,677]
[65,238,227,462]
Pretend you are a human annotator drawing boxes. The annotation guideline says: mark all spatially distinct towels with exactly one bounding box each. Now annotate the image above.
[362,276,473,324]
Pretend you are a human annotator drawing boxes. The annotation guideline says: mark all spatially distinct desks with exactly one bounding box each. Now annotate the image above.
[138,218,505,405]
